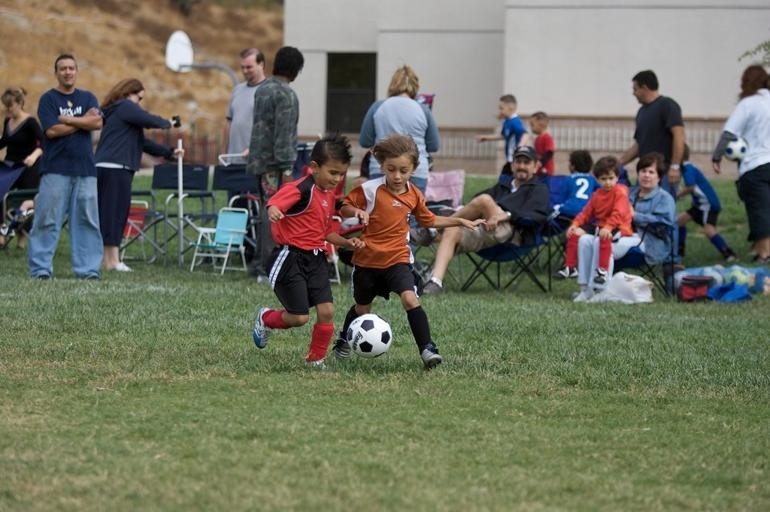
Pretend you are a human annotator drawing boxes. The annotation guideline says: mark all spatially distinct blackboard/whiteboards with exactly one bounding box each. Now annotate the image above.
[375,26,502,131]
[278,48,374,141]
[502,3,770,120]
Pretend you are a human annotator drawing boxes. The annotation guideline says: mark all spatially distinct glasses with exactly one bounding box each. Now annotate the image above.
[137,95,144,102]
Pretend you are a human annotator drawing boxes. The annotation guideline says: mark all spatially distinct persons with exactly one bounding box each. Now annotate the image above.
[478,94,530,176]
[28,53,107,281]
[617,69,685,269]
[553,150,601,240]
[359,65,440,266]
[558,155,633,284]
[0,86,45,251]
[221,47,269,265]
[572,151,675,298]
[408,145,549,297]
[713,65,769,264]
[529,111,556,177]
[678,143,738,262]
[332,134,487,370]
[251,135,366,372]
[94,78,185,273]
[243,46,304,276]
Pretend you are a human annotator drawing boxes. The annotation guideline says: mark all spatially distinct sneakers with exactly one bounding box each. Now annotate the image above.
[416,279,445,297]
[303,356,334,377]
[673,250,770,271]
[252,307,277,350]
[419,341,443,370]
[0,236,6,249]
[407,227,436,247]
[552,264,579,281]
[16,239,25,251]
[104,260,135,274]
[593,266,610,284]
[332,330,354,364]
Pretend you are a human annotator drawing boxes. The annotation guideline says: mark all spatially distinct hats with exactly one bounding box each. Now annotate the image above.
[513,145,537,161]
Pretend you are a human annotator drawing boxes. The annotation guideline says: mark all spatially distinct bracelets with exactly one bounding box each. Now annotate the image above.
[670,163,680,169]
[506,211,511,220]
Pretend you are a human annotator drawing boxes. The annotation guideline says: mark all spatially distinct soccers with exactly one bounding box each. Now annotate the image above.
[346,314,392,359]
